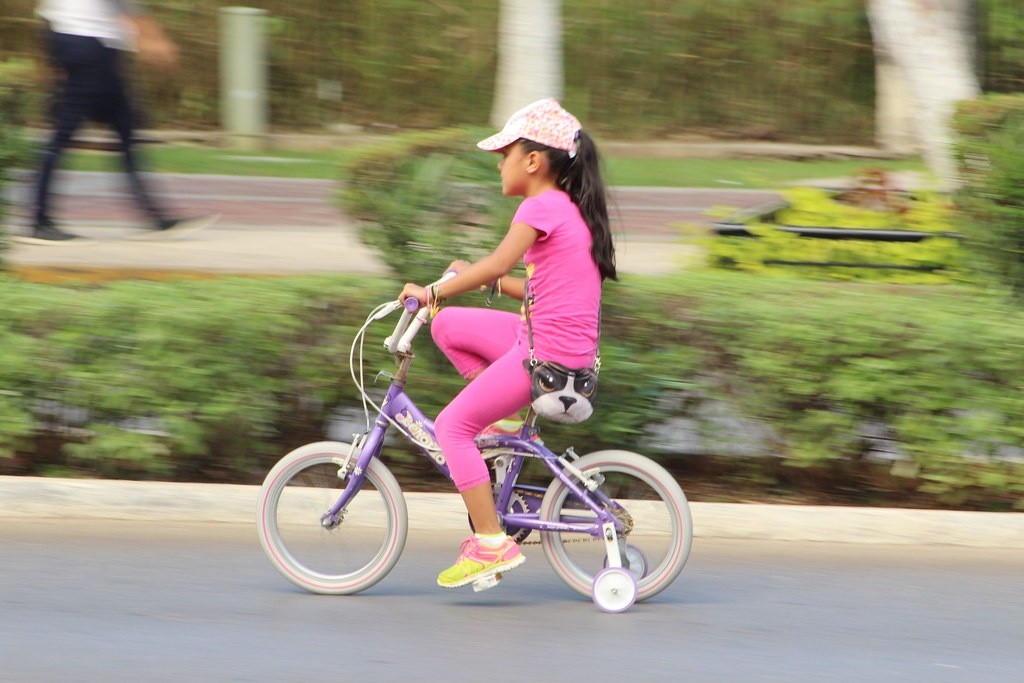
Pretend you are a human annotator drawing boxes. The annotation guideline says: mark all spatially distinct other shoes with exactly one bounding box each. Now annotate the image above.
[159,212,220,240]
[21,225,94,244]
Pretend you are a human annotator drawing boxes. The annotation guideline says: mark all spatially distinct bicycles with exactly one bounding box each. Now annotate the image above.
[253,269,695,614]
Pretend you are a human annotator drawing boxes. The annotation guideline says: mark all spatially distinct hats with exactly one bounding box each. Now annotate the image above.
[478,96,579,158]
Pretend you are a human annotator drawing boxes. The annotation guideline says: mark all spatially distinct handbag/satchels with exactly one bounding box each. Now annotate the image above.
[531,360,597,422]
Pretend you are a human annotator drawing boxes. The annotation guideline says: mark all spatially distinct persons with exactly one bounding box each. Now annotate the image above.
[24,1,215,244]
[397,99,622,588]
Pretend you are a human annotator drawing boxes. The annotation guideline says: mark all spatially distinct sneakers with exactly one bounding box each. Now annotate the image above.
[475,421,544,458]
[436,536,527,589]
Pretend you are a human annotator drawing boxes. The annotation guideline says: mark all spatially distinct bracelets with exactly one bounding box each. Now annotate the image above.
[422,284,443,307]
[479,277,501,305]
